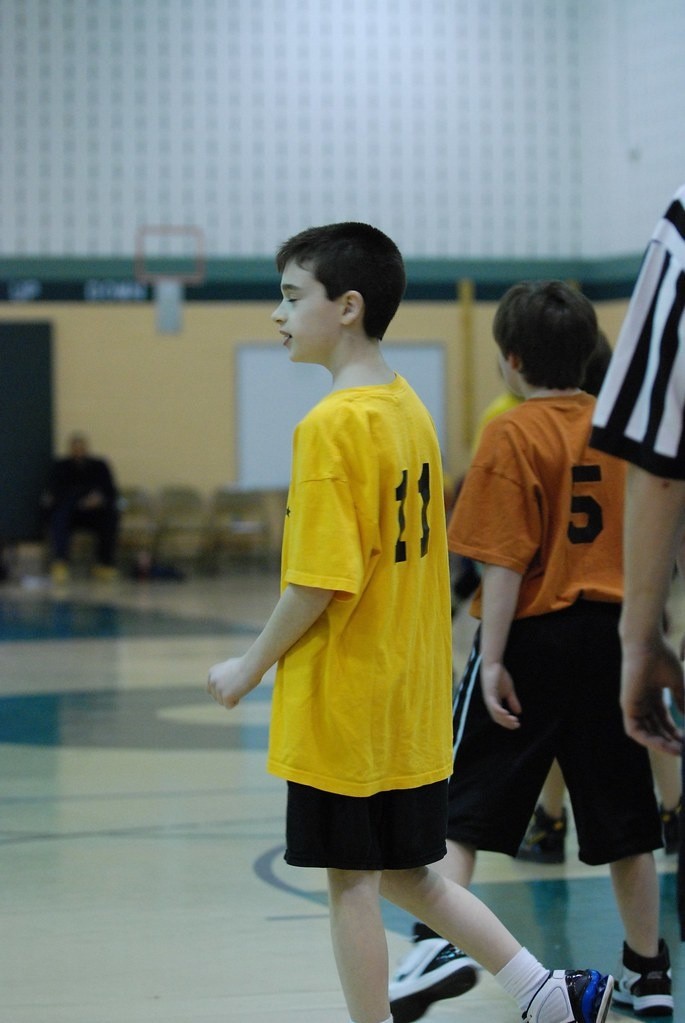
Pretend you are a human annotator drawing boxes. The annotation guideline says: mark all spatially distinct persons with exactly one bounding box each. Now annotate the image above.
[585,184,685,940]
[203,221,616,1023]
[46,434,119,587]
[473,327,678,869]
[388,278,675,1023]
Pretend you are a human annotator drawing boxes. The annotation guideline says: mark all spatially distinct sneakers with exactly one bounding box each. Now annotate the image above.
[514,803,568,867]
[659,792,685,856]
[49,560,73,587]
[386,922,481,1023]
[611,936,675,1017]
[519,967,616,1023]
[90,563,123,583]
